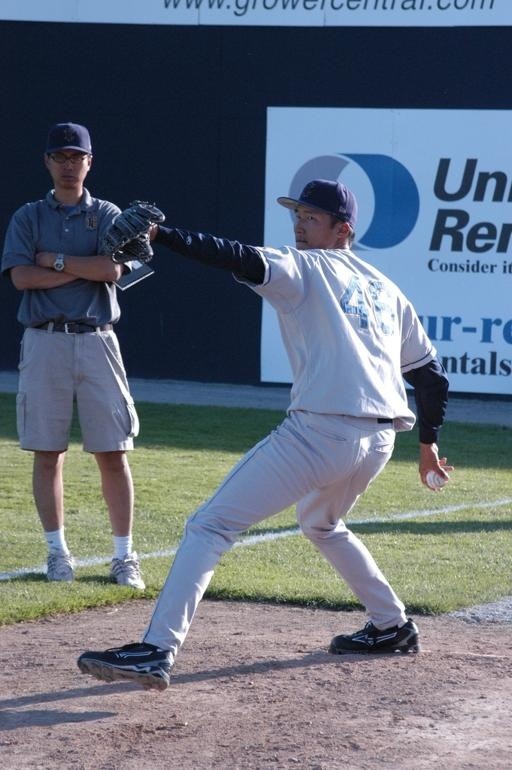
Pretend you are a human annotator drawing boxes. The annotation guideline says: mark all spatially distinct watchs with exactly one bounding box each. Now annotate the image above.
[54,253,64,272]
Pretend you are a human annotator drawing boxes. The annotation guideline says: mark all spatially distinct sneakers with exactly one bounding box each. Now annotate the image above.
[326,616,422,657]
[75,642,177,692]
[45,551,75,585]
[107,550,148,593]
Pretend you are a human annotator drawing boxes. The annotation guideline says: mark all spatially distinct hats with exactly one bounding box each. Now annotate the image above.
[274,176,360,228]
[42,121,95,156]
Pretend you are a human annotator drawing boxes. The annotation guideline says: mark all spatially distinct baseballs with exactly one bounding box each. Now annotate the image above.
[426,470,448,489]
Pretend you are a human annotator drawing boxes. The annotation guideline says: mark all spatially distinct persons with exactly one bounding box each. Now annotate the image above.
[77,180,454,689]
[2,123,145,592]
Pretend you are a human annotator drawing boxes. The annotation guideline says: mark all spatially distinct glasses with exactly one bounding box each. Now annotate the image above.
[46,152,89,164]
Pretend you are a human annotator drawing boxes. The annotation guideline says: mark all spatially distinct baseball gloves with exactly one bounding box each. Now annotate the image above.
[102,200,166,264]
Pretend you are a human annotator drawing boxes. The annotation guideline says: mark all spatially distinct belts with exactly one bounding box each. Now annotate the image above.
[32,318,115,335]
